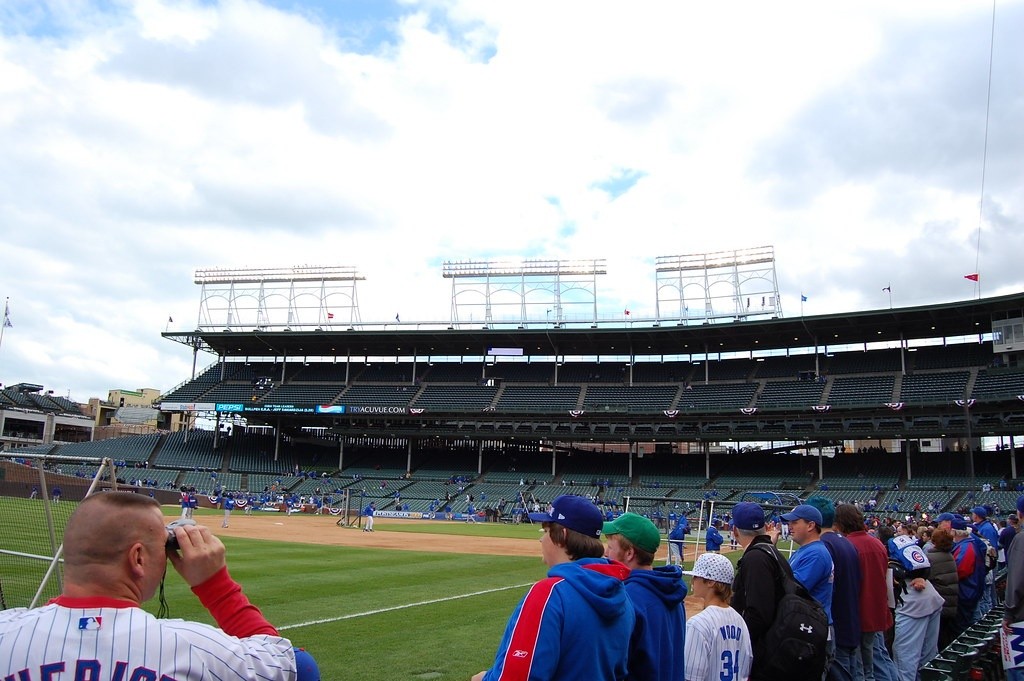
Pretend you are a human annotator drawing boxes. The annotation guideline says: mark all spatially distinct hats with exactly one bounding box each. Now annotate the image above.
[1016,496,1024,513]
[951,518,967,530]
[1008,514,1015,519]
[682,553,734,584]
[779,505,822,526]
[602,512,661,554]
[933,512,955,522]
[953,514,964,520]
[970,507,987,518]
[686,526,691,535]
[712,518,719,525]
[528,495,603,539]
[803,496,834,527]
[728,501,765,530]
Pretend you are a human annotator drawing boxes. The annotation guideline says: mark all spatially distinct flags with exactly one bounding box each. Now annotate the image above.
[964,274,978,281]
[328,313,334,318]
[802,295,807,301]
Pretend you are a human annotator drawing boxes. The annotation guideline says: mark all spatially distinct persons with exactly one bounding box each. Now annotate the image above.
[16,457,99,480]
[52,485,62,504]
[678,509,698,549]
[117,465,552,525]
[705,519,723,554]
[562,478,1024,529]
[30,485,38,499]
[0,491,320,681]
[117,457,149,469]
[666,523,691,566]
[728,518,737,550]
[364,502,376,532]
[222,494,234,528]
[469,494,1024,681]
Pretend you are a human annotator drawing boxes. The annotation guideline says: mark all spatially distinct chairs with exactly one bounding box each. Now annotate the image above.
[918,599,1004,681]
[0,340,1024,521]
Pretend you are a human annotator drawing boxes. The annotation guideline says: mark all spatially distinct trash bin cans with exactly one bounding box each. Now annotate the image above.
[16,458,20,463]
[20,458,25,464]
[26,460,31,465]
[324,507,329,514]
[724,523,729,531]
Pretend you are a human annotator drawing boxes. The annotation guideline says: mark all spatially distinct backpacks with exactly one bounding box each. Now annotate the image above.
[744,544,829,681]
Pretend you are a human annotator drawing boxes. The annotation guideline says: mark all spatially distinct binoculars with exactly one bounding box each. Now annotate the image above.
[165,518,196,550]
